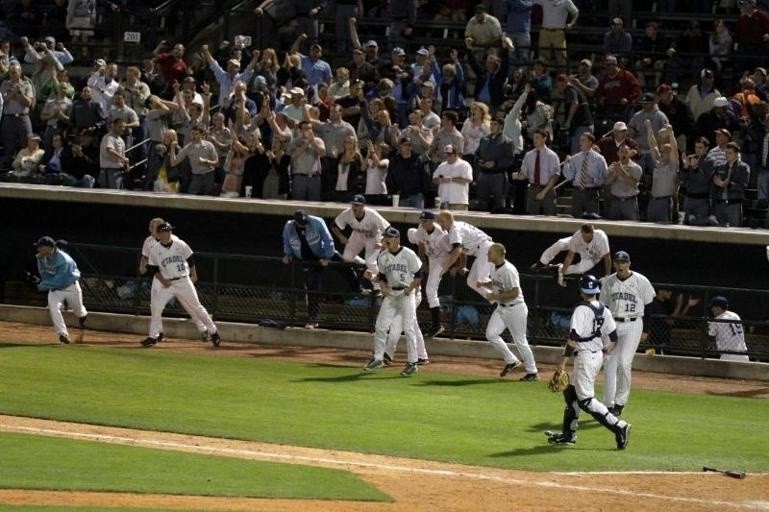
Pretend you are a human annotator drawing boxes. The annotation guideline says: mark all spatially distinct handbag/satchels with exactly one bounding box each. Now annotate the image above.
[223,138,239,173]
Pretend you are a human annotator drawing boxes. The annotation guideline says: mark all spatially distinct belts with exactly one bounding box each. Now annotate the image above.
[614,317,636,322]
[392,287,404,290]
[613,196,636,202]
[651,195,671,200]
[292,174,321,177]
[541,28,565,32]
[499,303,513,308]
[4,113,29,117]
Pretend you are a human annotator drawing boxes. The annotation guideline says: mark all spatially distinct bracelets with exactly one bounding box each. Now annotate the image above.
[560,343,575,357]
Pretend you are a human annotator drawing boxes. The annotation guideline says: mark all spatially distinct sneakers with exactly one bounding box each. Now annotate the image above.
[141,337,156,345]
[371,290,382,296]
[60,334,71,344]
[425,325,444,337]
[400,362,418,376]
[363,360,384,372]
[520,373,540,381]
[530,262,547,272]
[79,316,88,327]
[548,437,576,446]
[211,332,220,346]
[501,360,522,376]
[361,288,371,295]
[306,322,319,328]
[158,333,163,342]
[417,358,429,365]
[548,263,562,270]
[383,358,392,366]
[202,331,212,341]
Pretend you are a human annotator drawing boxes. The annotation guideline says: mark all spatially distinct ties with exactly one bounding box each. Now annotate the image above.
[534,151,540,189]
[579,154,588,192]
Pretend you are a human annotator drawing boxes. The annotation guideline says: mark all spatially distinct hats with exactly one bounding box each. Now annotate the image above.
[713,97,730,107]
[227,58,240,68]
[580,275,601,294]
[157,223,176,231]
[443,144,457,153]
[605,56,617,64]
[391,47,406,56]
[401,137,414,145]
[351,194,366,205]
[637,93,655,105]
[367,40,378,48]
[580,59,593,67]
[614,121,627,131]
[93,59,107,66]
[352,48,365,54]
[610,18,623,26]
[289,87,305,97]
[421,81,435,92]
[708,296,728,309]
[381,227,400,237]
[294,210,310,225]
[311,44,322,51]
[615,251,630,262]
[714,129,731,137]
[701,69,711,78]
[44,37,56,43]
[419,211,434,219]
[416,49,430,57]
[33,236,55,248]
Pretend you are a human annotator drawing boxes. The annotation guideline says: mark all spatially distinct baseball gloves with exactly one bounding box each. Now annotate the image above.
[548,369,569,393]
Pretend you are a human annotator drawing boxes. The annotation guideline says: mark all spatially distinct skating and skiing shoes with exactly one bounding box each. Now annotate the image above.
[620,424,632,448]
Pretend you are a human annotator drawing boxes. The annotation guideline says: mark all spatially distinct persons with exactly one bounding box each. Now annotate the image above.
[283,209,335,329]
[438,209,496,309]
[321,249,363,302]
[34,236,88,345]
[545,274,632,450]
[706,296,750,362]
[363,237,431,366]
[529,224,612,287]
[476,242,541,380]
[408,211,469,338]
[138,217,213,343]
[523,0,768,231]
[328,1,561,208]
[141,221,221,347]
[1,1,329,201]
[330,194,392,296]
[598,250,656,415]
[648,288,701,355]
[363,227,424,377]
